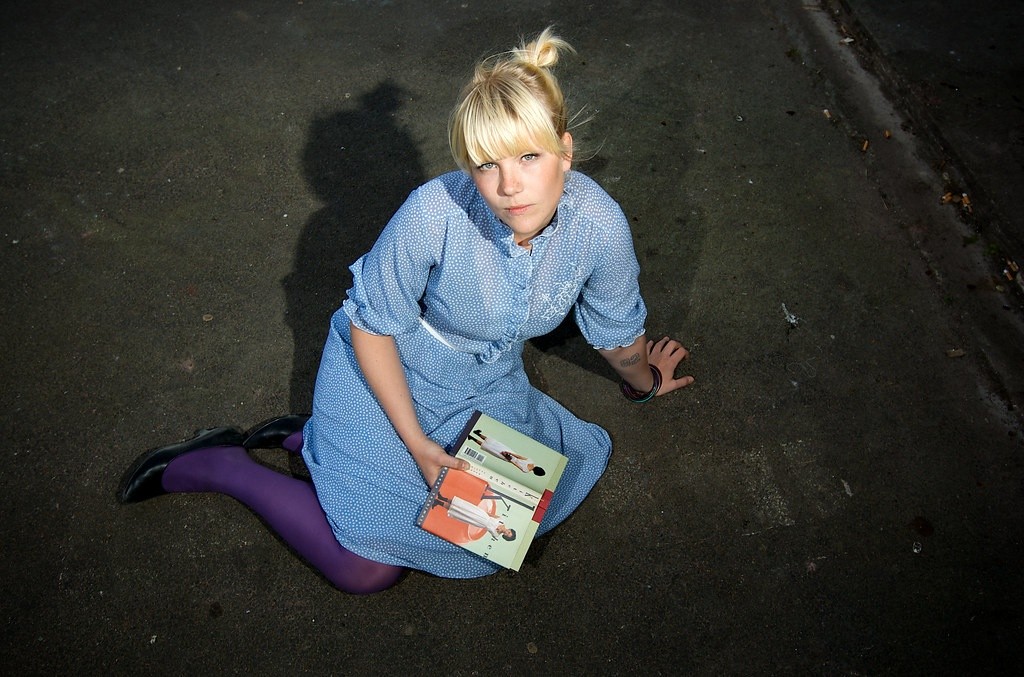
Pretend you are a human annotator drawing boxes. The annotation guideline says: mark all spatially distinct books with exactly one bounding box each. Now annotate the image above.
[416,410,570,572]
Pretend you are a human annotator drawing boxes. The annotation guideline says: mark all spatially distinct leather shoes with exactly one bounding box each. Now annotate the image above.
[241,415,310,455]
[120,426,240,501]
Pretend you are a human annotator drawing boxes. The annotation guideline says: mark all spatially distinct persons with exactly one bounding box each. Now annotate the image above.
[468,430,545,476]
[119,28,695,594]
[432,492,516,541]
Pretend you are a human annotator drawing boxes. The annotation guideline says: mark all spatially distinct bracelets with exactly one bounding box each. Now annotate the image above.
[619,364,662,402]
